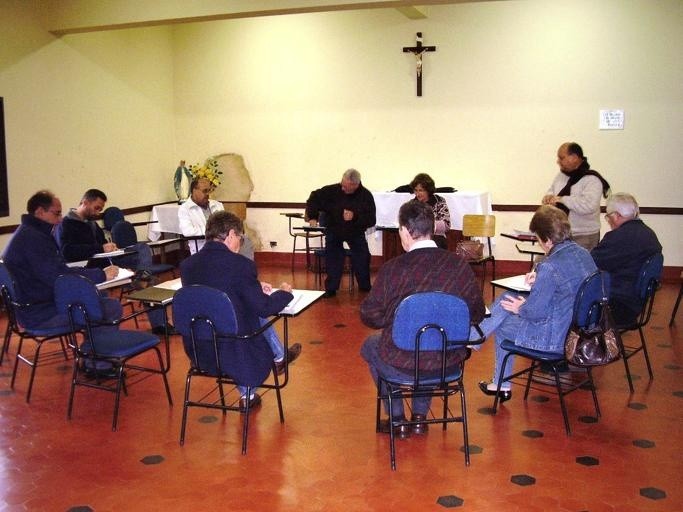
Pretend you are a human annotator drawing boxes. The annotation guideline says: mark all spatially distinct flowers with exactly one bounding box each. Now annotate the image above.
[187,158,224,194]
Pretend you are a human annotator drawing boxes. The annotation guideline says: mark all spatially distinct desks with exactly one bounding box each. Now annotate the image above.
[146,202,186,264]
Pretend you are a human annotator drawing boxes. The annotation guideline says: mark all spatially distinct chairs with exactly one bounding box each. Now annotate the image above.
[462,215,496,304]
[111,220,174,301]
[279,211,325,273]
[616,253,664,393]
[376,292,486,471]
[54,272,174,432]
[103,207,125,232]
[492,271,611,436]
[0,259,86,403]
[171,284,289,456]
[303,224,355,296]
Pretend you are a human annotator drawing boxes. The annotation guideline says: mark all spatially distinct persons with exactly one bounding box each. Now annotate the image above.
[589,194,663,324]
[359,200,485,439]
[543,143,604,252]
[179,211,302,410]
[305,169,377,297]
[411,174,452,248]
[179,179,253,261]
[467,205,601,403]
[55,188,174,334]
[1,190,126,377]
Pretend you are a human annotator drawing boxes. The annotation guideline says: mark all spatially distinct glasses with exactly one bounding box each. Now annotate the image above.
[198,186,213,195]
[236,232,244,245]
[48,207,64,217]
[604,210,624,221]
[413,187,428,194]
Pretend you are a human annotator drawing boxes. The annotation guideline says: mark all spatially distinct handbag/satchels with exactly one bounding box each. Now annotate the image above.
[565,270,623,368]
[456,239,483,260]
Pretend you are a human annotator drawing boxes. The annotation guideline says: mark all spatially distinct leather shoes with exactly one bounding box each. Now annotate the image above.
[83,365,127,381]
[359,285,371,292]
[377,418,409,441]
[76,355,93,373]
[539,361,570,372]
[151,322,182,337]
[239,394,262,414]
[272,342,302,375]
[324,290,337,299]
[131,270,153,283]
[410,415,430,434]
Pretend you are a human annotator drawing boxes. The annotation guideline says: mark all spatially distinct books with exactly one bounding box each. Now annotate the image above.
[266,286,301,311]
[94,249,124,256]
[504,274,533,290]
[94,265,134,287]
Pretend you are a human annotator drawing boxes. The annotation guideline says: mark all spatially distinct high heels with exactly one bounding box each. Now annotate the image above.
[479,378,511,404]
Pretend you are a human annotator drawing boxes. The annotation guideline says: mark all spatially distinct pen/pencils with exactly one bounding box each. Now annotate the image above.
[108,237,111,242]
[107,256,114,266]
[529,263,537,279]
